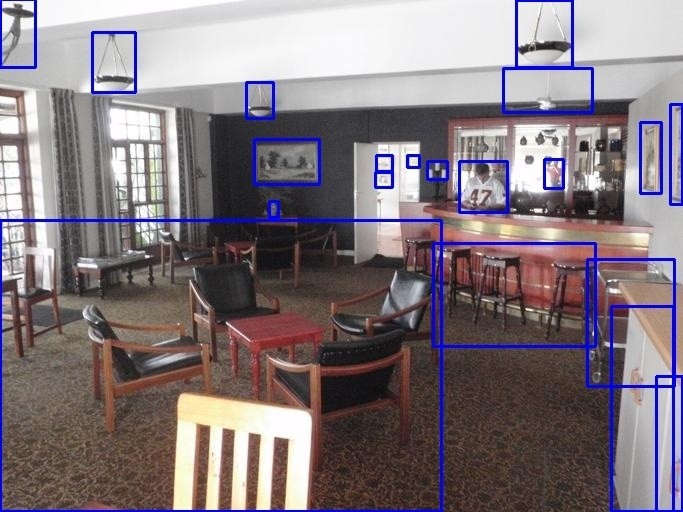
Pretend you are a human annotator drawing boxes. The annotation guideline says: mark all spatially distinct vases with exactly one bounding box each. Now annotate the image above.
[269,201,278,218]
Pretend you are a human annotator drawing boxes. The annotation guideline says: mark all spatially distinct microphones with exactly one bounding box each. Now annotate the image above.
[433,243,595,346]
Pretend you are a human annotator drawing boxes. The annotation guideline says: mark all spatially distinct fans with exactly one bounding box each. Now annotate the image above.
[504,68,593,112]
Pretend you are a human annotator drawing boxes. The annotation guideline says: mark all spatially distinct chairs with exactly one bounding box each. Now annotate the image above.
[265,328,411,442]
[190,262,281,354]
[293,221,337,267]
[2,247,63,348]
[329,268,438,367]
[173,393,318,511]
[83,304,212,433]
[241,224,300,290]
[158,230,218,284]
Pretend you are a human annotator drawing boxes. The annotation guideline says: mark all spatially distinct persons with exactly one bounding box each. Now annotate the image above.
[460,162,507,212]
[544,159,564,188]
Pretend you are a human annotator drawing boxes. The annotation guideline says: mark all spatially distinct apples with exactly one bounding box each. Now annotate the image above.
[427,161,447,180]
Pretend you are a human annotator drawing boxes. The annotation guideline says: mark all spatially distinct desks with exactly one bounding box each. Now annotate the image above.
[225,241,251,262]
[226,313,326,399]
[0,277,23,356]
[76,253,155,299]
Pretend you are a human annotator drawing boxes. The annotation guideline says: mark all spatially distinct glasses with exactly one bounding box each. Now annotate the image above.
[516,0,572,67]
[247,82,274,118]
[0,0,35,68]
[93,33,135,92]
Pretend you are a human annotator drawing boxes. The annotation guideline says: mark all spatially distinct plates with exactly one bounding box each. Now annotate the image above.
[611,306,674,512]
[656,376,683,512]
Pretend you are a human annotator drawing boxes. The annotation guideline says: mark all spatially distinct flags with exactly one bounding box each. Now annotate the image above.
[269,201,278,218]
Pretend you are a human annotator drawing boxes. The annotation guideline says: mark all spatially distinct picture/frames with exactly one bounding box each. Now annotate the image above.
[376,173,393,188]
[376,156,393,171]
[641,123,662,193]
[254,140,319,185]
[408,156,420,167]
[670,105,683,204]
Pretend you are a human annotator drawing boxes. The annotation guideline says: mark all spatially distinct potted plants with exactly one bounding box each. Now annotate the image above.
[641,123,662,193]
[376,156,393,171]
[376,173,393,188]
[670,105,683,204]
[408,156,420,167]
[254,140,319,185]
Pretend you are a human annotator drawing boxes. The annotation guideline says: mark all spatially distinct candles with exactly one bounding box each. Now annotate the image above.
[427,161,447,180]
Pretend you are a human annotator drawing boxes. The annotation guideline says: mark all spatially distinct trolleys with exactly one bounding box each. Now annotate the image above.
[504,68,593,112]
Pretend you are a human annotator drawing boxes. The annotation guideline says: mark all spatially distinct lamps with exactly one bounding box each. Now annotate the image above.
[247,82,274,118]
[93,33,135,92]
[516,0,572,67]
[0,0,35,68]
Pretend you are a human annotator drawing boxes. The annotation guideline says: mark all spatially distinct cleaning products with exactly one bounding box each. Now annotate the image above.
[588,260,674,386]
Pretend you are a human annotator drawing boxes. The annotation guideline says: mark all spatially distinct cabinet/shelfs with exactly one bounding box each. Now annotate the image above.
[656,376,683,512]
[611,306,674,512]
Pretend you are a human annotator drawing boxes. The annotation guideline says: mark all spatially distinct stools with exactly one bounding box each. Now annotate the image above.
[433,243,595,346]
